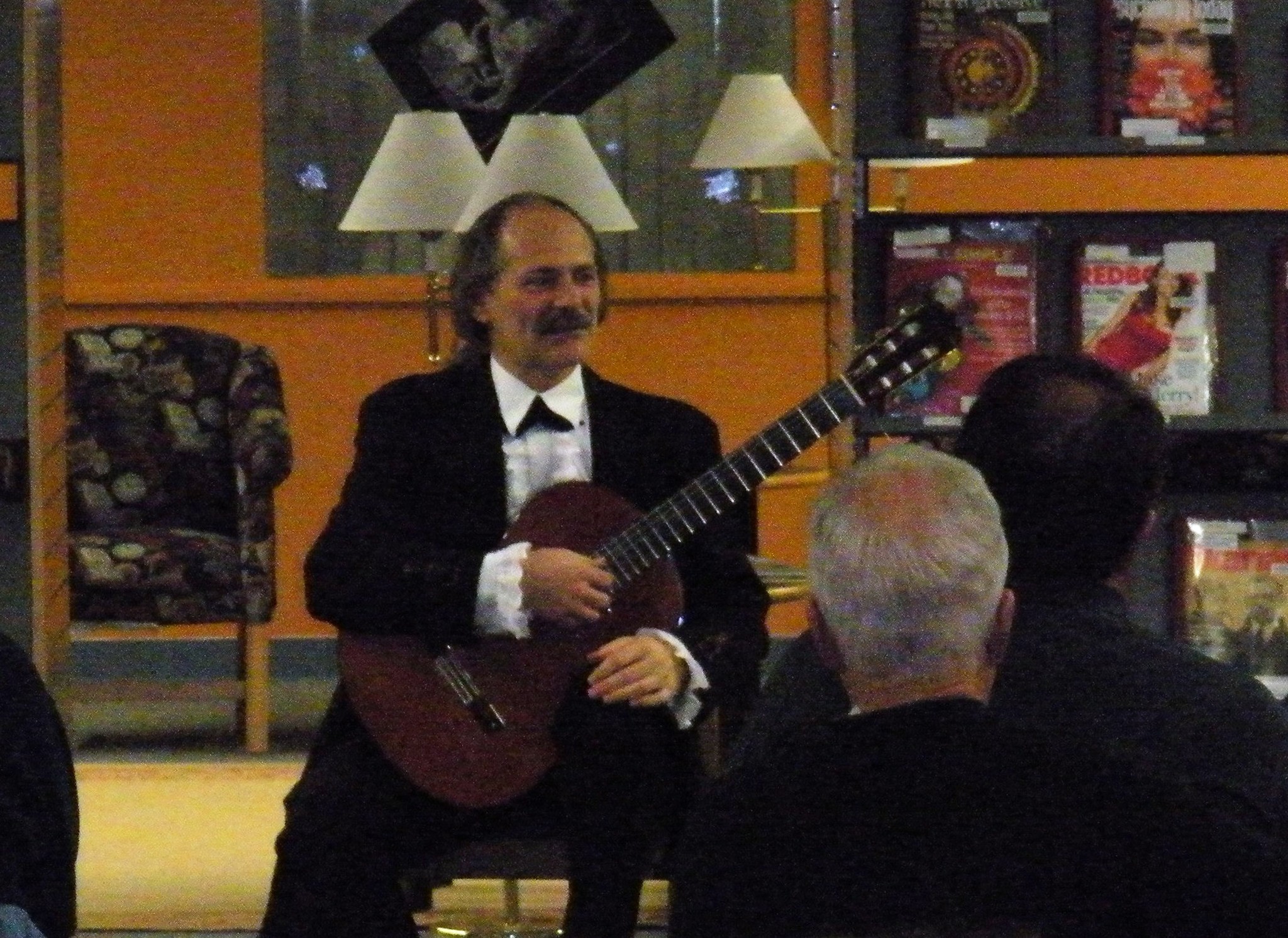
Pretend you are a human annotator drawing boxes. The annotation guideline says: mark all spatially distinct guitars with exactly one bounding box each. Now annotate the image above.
[337,298,963,808]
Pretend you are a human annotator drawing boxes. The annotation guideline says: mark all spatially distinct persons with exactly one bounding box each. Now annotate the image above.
[1077,254,1200,415]
[1112,0,1237,143]
[662,446,1254,937]
[727,349,1282,793]
[259,193,773,938]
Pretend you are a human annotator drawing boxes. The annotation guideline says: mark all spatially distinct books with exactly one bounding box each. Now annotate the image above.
[904,0,1063,156]
[1087,0,1240,149]
[1176,515,1288,679]
[879,205,1045,422]
[1080,241,1219,416]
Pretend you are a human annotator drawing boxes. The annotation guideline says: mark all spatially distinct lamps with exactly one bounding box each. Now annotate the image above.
[338,112,639,365]
[689,74,834,214]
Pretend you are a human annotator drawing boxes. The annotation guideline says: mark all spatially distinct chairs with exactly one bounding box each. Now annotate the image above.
[63,324,292,756]
[437,677,761,938]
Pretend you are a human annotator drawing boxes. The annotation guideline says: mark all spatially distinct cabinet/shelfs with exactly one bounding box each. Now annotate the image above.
[856,142,1287,432]
